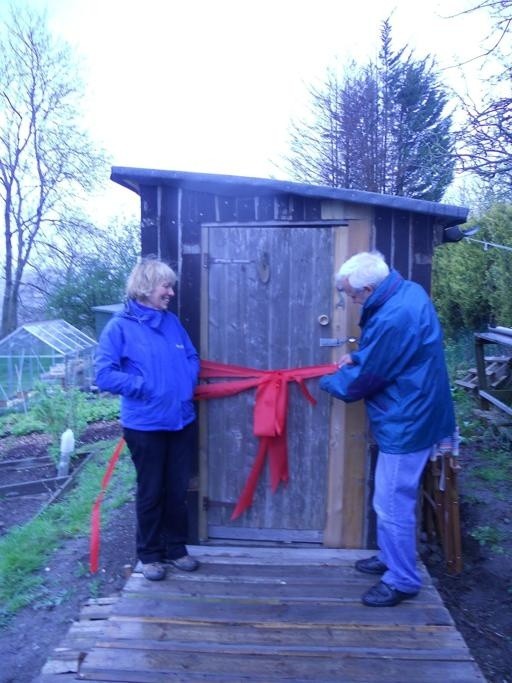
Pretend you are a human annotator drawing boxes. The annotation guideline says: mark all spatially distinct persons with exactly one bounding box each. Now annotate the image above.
[92,259,200,580]
[319,252,456,606]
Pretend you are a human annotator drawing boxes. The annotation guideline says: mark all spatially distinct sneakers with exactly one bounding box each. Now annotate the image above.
[355,555,389,574]
[361,582,419,607]
[141,555,197,581]
